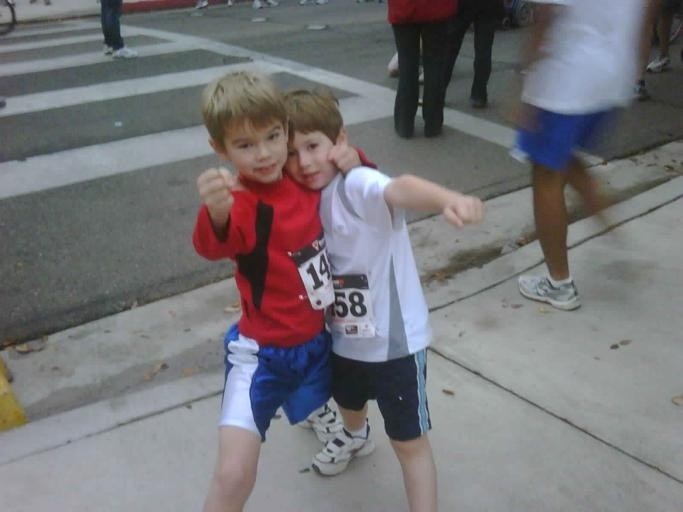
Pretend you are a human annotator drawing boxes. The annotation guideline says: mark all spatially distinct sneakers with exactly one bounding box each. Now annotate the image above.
[110,45,139,59]
[250,0,264,10]
[261,0,279,8]
[631,80,649,102]
[103,42,114,56]
[309,417,377,477]
[299,0,329,7]
[296,403,346,448]
[518,273,583,312]
[645,53,672,74]
[196,0,237,9]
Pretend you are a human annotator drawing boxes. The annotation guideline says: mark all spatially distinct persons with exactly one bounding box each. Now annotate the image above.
[386,0,504,141]
[99,1,139,60]
[190,67,379,512]
[500,1,659,313]
[279,89,488,512]
